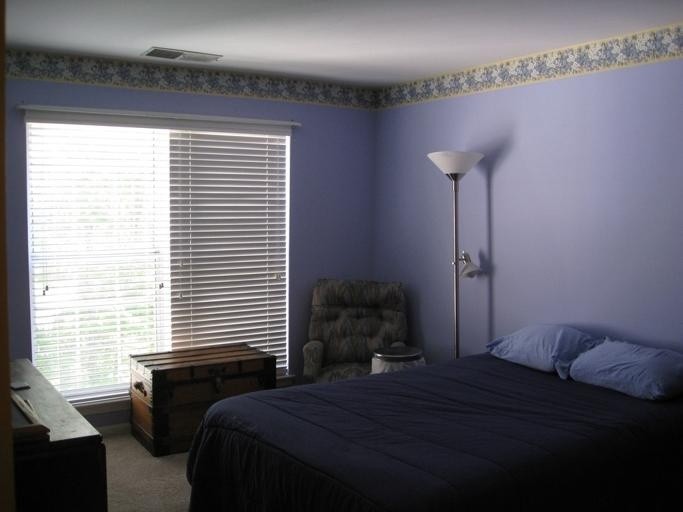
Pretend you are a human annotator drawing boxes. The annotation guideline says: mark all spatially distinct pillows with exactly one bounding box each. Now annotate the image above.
[486,324,604,380]
[570,335,683,401]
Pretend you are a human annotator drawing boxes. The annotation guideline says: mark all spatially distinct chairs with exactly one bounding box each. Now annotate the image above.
[303,277,409,384]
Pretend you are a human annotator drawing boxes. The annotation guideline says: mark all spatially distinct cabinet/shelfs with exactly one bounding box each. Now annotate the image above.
[128,342,277,458]
[8,358,107,512]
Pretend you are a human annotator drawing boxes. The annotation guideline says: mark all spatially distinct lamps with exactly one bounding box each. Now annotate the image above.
[428,150,485,365]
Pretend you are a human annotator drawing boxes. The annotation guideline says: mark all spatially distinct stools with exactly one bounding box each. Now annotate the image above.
[371,346,426,374]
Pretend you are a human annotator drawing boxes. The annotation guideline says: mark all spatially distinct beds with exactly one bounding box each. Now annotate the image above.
[187,353,683,512]
